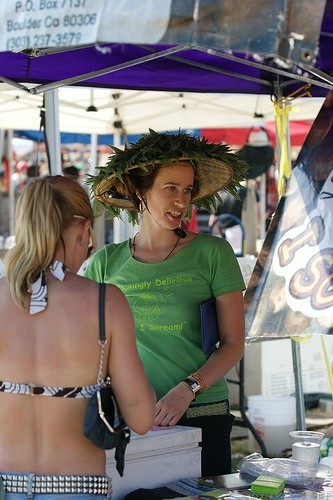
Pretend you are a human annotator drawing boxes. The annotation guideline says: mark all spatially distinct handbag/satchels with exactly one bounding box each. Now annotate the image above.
[83,283,130,477]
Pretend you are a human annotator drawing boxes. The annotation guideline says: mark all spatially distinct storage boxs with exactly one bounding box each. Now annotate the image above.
[105,423,202,500]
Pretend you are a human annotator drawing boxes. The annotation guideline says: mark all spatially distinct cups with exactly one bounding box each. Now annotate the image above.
[288,430,324,464]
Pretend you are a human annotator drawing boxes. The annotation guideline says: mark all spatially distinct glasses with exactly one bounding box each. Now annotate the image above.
[73,215,97,259]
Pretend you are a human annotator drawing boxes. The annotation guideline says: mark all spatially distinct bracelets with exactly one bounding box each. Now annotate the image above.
[181,373,206,395]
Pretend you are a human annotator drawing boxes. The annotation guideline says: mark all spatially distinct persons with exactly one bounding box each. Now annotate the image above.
[0,175,158,500]
[81,128,251,478]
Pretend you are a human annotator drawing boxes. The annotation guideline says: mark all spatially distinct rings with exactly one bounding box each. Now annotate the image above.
[165,416,171,420]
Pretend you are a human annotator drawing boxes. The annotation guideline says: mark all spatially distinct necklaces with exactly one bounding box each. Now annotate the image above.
[133,228,183,262]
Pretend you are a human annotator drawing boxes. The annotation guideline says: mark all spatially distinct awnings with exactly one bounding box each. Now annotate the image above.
[0,80,325,167]
[0,41,333,162]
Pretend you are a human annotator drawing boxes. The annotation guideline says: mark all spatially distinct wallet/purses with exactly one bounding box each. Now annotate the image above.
[200,298,220,355]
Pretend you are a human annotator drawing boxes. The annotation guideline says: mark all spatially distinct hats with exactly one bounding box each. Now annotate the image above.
[83,127,253,226]
